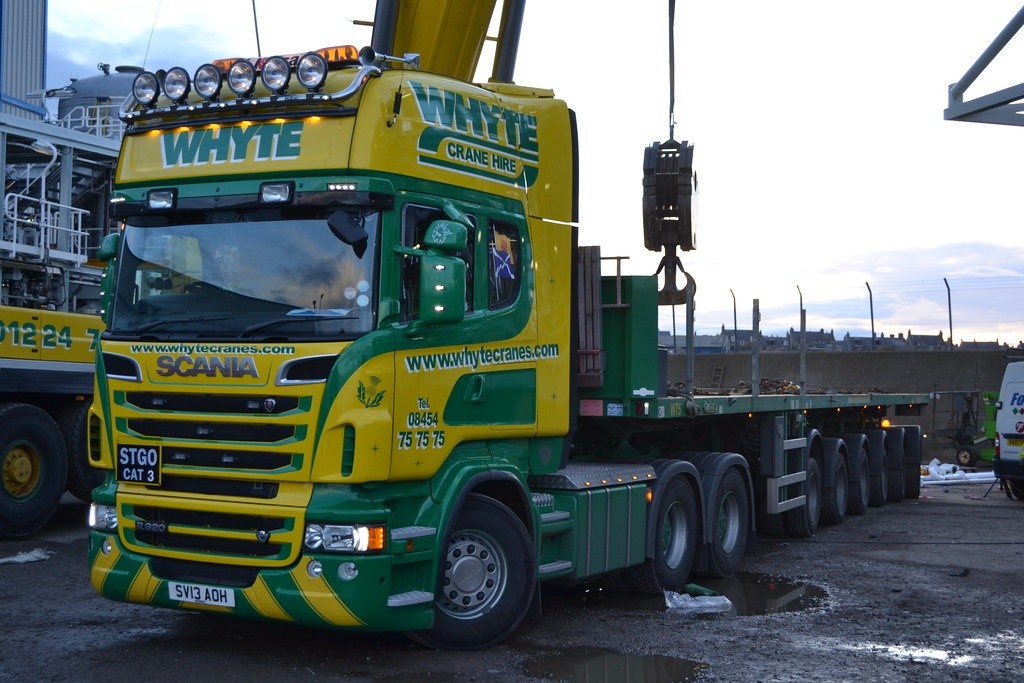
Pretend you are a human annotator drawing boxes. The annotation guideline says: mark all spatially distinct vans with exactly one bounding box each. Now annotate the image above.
[993,362,1024,501]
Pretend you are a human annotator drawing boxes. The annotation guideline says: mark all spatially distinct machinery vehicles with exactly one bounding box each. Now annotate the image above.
[933,389,999,466]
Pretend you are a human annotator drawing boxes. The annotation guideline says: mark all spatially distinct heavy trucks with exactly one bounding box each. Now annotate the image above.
[88,46,937,640]
[0,103,129,538]
